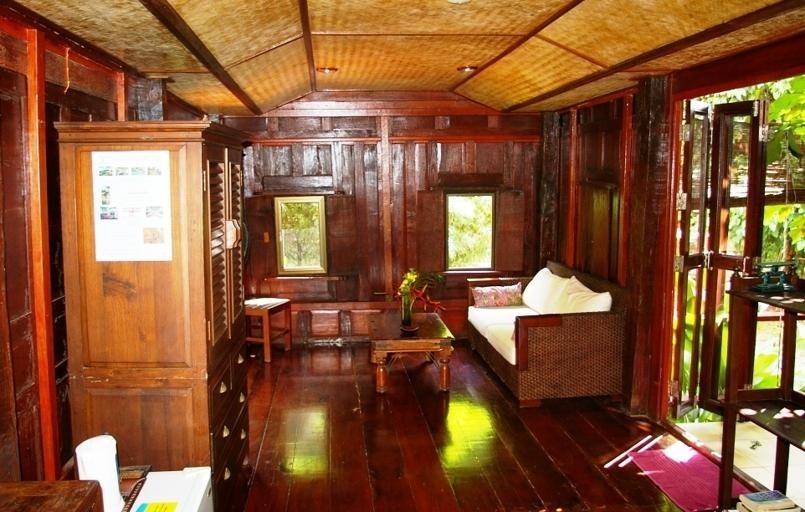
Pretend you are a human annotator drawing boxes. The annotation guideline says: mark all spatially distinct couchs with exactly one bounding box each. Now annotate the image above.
[463,259,631,409]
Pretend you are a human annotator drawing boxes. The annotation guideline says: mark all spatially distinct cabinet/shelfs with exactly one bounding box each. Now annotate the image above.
[41,117,262,512]
[715,273,805,512]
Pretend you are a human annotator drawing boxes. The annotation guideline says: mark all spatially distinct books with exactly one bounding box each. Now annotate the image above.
[736,490,801,512]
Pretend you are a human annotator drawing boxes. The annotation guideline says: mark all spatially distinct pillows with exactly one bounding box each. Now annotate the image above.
[466,267,613,324]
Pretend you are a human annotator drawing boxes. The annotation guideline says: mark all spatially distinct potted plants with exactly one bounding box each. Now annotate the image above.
[398,268,419,326]
[420,272,447,298]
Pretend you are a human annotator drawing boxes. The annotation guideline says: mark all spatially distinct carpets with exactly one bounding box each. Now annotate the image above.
[625,446,752,512]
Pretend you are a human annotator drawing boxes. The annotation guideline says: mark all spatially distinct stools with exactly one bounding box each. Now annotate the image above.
[242,297,292,364]
